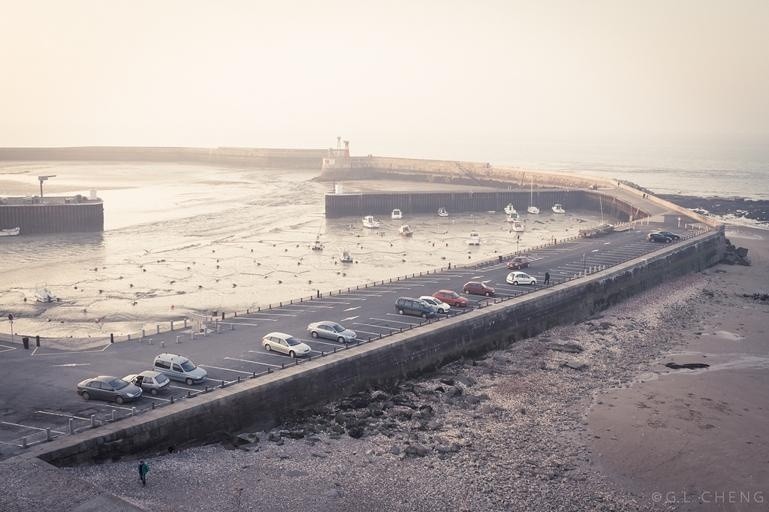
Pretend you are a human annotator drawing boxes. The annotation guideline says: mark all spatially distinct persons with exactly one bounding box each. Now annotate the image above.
[543,271,551,285]
[138,460,150,487]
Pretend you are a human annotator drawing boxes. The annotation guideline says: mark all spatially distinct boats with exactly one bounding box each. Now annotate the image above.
[398,224,413,236]
[340,251,354,263]
[312,239,323,251]
[551,203,566,214]
[391,208,402,220]
[468,230,481,245]
[504,202,525,232]
[0,228,21,239]
[527,206,541,215]
[437,207,449,216]
[361,215,380,227]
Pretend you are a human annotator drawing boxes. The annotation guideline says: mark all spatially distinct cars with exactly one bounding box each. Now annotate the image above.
[75,352,208,405]
[647,231,683,244]
[506,271,538,286]
[464,282,496,298]
[261,331,312,358]
[305,320,358,344]
[396,290,468,319]
[506,255,531,269]
